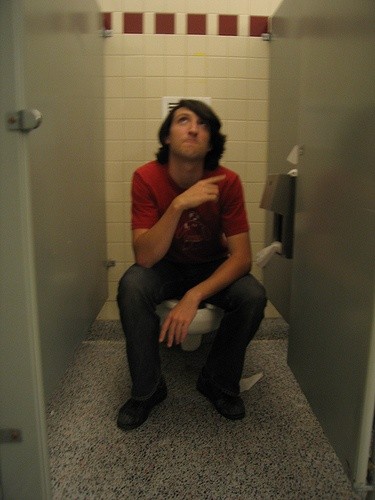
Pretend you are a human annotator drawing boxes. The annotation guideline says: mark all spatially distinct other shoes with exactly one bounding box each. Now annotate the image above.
[117,376,167,430]
[196,377,246,420]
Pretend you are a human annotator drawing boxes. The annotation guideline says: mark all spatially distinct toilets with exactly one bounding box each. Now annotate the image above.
[150,293,226,353]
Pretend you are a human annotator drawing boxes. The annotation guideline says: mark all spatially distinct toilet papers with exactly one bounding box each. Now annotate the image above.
[255,240,288,273]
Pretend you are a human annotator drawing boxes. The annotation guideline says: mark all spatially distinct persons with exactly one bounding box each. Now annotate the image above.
[117,100,266,429]
